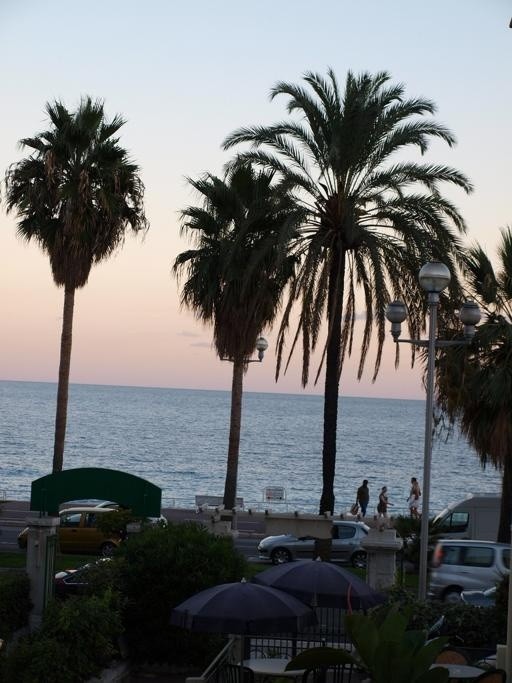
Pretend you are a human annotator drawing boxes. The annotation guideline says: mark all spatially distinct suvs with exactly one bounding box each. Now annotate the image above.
[427,539,512,603]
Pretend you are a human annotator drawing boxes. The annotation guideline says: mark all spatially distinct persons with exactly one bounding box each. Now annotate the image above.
[376,484,394,519]
[355,478,372,518]
[406,476,422,515]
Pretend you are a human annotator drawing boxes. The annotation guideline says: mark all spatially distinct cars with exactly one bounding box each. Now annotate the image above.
[66,501,168,533]
[17,507,128,557]
[257,521,404,568]
[459,587,496,608]
[54,557,111,594]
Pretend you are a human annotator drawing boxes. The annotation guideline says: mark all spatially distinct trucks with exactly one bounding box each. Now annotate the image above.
[407,492,502,551]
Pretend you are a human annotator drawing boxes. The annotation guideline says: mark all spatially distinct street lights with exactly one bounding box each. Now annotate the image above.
[214,337,268,365]
[387,256,482,601]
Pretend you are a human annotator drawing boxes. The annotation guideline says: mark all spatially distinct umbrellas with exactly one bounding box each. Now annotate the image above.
[253,552,373,649]
[167,576,318,680]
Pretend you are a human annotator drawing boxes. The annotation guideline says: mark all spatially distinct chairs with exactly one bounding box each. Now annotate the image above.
[216,664,256,681]
[433,650,469,665]
[301,658,356,683]
[476,669,507,682]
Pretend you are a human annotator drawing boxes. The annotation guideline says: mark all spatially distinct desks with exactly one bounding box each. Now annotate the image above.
[428,663,486,682]
[235,656,359,682]
